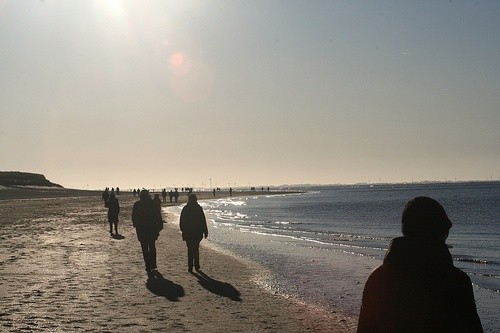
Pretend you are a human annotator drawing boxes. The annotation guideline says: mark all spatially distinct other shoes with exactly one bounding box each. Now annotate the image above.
[195,265,200,270]
[188,266,192,272]
[151,265,157,269]
[145,267,151,271]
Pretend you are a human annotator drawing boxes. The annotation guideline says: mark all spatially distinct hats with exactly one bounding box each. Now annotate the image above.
[402,196,452,242]
[188,193,197,202]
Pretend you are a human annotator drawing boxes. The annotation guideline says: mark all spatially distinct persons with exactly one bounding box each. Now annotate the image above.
[262,187,263,192]
[181,187,193,193]
[162,188,167,202]
[105,192,120,234]
[179,194,209,272]
[137,188,140,196]
[268,187,269,192]
[213,188,216,197]
[101,187,120,203]
[230,187,232,196]
[132,190,163,271]
[169,190,173,203]
[356,197,485,333]
[133,189,136,196]
[174,188,179,203]
[153,194,162,213]
